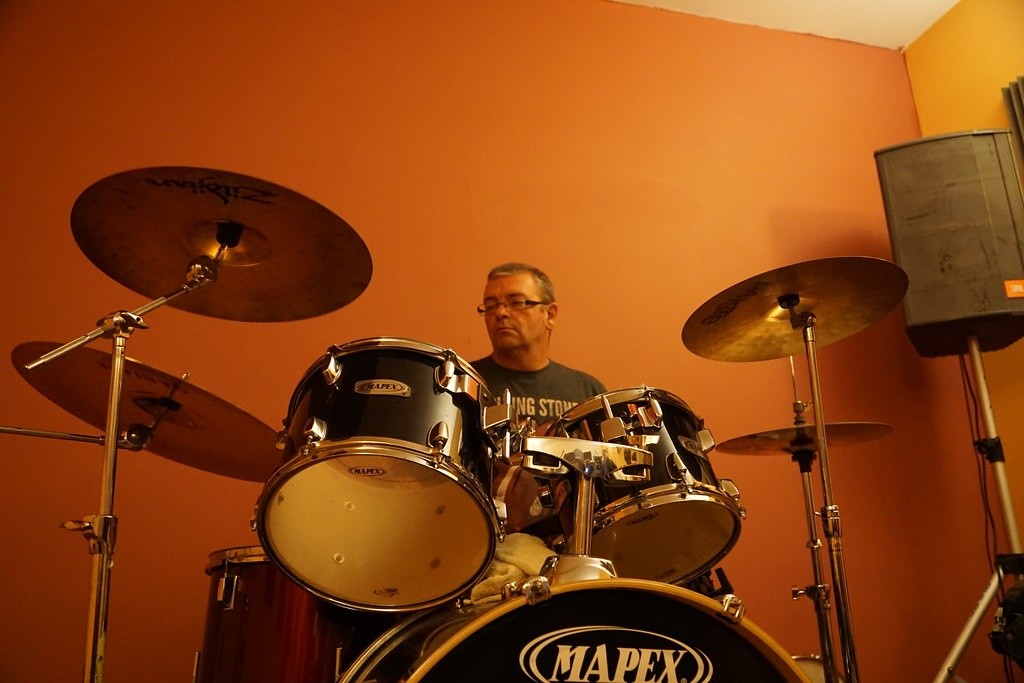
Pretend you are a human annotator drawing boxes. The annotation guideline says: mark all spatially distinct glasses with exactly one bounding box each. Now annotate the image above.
[477,298,548,317]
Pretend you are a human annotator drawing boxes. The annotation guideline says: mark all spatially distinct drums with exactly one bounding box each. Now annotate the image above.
[790,654,846,683]
[336,577,814,683]
[254,335,498,614]
[192,546,366,683]
[533,384,743,586]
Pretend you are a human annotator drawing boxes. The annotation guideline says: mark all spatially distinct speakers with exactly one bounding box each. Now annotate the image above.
[872,127,1024,357]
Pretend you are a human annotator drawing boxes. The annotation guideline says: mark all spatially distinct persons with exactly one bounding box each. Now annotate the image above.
[461,263,609,538]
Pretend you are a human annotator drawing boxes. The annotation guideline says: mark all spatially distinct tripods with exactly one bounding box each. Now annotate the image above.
[932,334,1024,683]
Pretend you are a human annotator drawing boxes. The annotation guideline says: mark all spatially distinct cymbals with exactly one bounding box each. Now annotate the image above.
[10,341,277,484]
[715,421,896,457]
[680,254,910,364]
[69,165,374,322]
